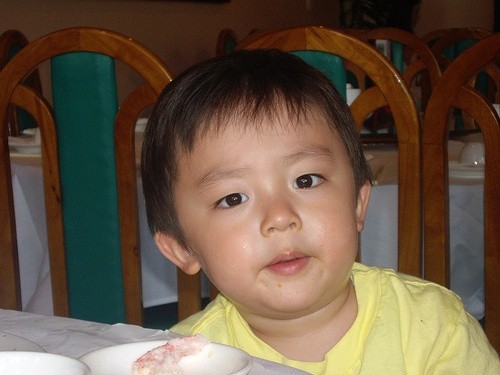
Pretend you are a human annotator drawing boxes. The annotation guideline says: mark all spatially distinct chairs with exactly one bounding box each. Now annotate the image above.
[212,19,499,351]
[0,29,45,136]
[0,27,203,329]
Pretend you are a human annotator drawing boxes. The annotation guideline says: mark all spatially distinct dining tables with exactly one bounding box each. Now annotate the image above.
[3,133,485,325]
[0,305,314,375]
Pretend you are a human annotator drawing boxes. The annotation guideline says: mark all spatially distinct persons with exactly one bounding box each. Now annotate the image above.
[138,47,499,375]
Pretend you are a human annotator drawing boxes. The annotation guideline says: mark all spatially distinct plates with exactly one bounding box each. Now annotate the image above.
[0,351,91,375]
[76,339,253,375]
